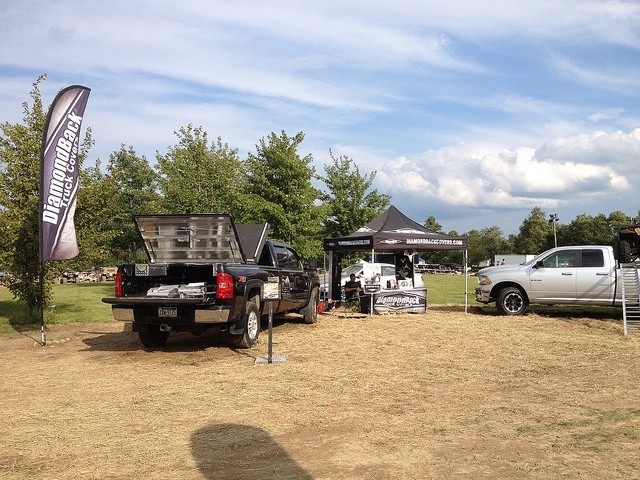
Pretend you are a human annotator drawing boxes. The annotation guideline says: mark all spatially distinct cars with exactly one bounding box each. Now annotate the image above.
[316,262,426,301]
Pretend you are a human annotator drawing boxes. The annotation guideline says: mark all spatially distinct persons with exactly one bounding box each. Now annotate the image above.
[501,258,506,265]
[343,273,360,300]
[496,260,500,266]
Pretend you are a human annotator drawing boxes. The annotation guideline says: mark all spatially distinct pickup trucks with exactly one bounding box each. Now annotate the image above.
[475,243,640,316]
[100,212,322,351]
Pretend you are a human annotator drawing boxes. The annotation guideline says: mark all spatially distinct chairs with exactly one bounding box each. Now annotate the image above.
[346,280,361,312]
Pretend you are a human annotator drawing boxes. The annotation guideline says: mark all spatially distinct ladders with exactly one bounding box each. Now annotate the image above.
[620,263,640,336]
